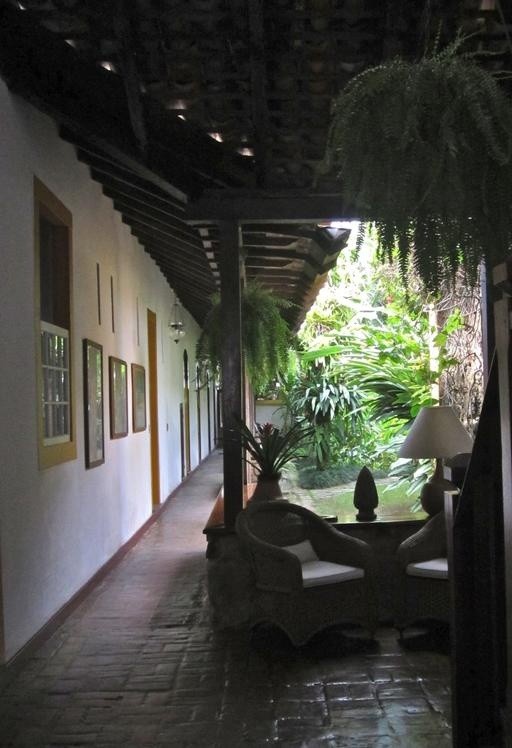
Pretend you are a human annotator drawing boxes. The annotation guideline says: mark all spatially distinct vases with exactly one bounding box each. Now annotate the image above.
[252,467,285,502]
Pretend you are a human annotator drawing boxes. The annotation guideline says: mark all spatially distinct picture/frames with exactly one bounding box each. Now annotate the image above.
[82,335,107,472]
[129,361,148,434]
[107,355,130,440]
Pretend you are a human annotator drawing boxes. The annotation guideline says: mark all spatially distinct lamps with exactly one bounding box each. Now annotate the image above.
[168,294,187,346]
[391,404,476,525]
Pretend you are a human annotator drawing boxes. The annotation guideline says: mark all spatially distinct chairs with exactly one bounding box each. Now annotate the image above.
[235,496,382,655]
[394,501,450,648]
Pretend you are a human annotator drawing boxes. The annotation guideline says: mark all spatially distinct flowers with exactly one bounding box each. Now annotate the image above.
[208,410,318,473]
